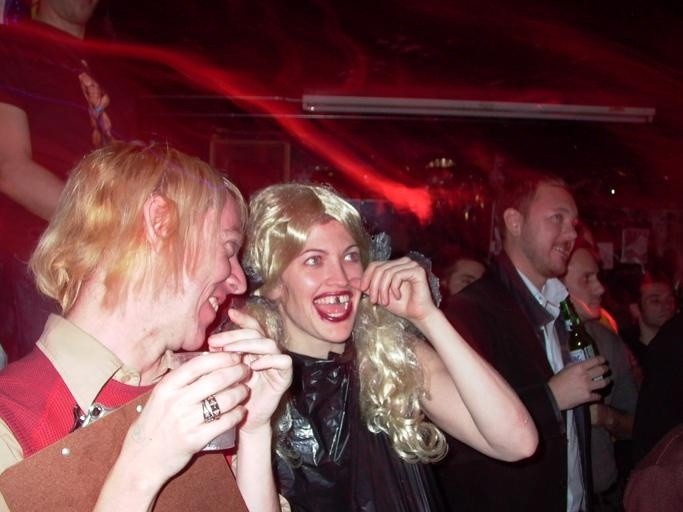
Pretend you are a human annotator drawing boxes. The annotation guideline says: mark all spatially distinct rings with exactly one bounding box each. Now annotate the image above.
[206,396,221,420]
[200,400,213,423]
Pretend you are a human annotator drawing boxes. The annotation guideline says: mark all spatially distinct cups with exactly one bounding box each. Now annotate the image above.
[169,350,239,454]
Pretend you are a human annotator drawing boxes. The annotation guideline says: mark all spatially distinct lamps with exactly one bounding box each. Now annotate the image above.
[303,0,656,126]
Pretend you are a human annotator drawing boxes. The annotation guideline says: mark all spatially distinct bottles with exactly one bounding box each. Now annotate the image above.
[558,298,605,394]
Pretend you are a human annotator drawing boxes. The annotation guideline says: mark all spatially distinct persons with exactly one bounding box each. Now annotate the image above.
[0,0,159,364]
[556,231,641,512]
[217,180,542,512]
[620,311,682,512]
[437,244,491,301]
[625,258,677,349]
[441,169,613,512]
[0,139,298,512]
